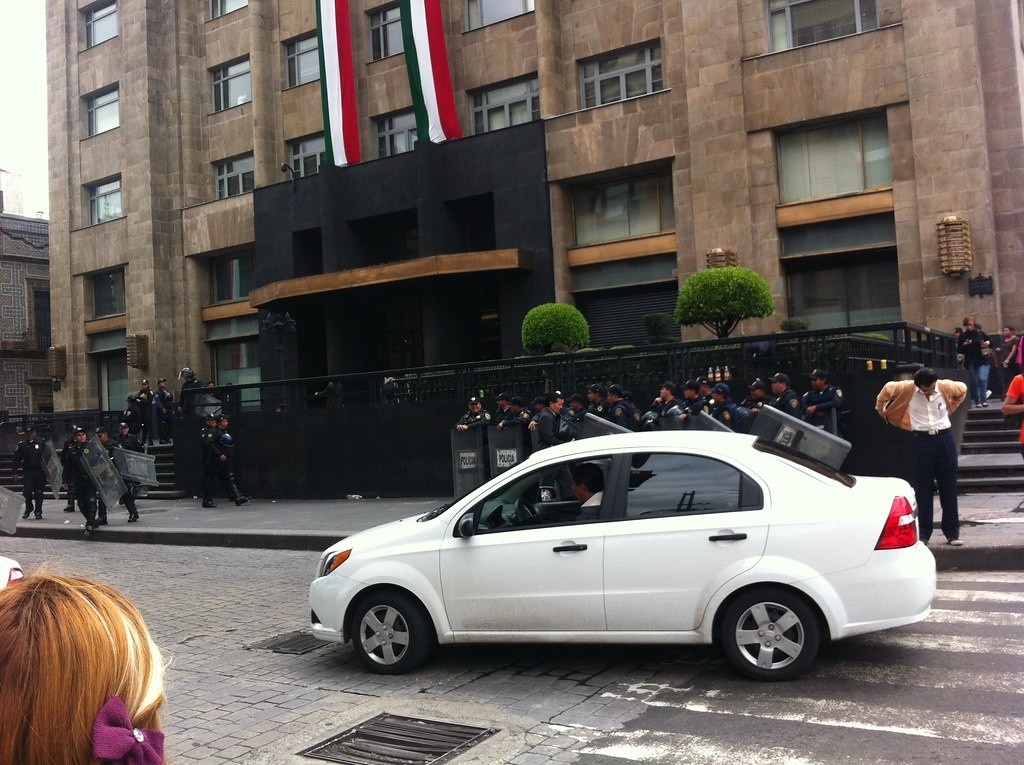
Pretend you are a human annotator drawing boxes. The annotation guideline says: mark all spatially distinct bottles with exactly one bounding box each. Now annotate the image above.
[347,494,362,500]
[708,365,729,381]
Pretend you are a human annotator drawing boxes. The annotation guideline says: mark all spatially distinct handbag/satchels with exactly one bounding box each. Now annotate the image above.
[977,330,992,358]
[556,417,578,441]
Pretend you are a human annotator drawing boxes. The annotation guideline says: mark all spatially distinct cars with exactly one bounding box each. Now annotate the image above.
[308,431,938,682]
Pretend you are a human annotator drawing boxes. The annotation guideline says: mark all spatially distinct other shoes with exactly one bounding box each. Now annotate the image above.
[235,495,252,506]
[23,507,42,520]
[202,499,216,509]
[129,513,139,522]
[97,517,108,525]
[948,540,963,545]
[85,519,98,530]
[976,403,988,407]
[63,505,76,512]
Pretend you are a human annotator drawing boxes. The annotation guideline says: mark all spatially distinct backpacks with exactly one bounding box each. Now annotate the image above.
[720,401,753,434]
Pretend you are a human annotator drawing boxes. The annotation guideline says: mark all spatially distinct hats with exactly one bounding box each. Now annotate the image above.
[205,413,226,424]
[808,369,825,379]
[70,423,128,436]
[747,381,765,389]
[126,395,137,404]
[680,377,730,397]
[566,393,585,401]
[468,393,545,405]
[141,378,167,391]
[24,425,35,434]
[588,384,633,401]
[768,373,790,384]
[659,381,675,390]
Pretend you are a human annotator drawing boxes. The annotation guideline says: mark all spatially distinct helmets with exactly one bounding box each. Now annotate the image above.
[179,367,197,378]
[220,433,236,448]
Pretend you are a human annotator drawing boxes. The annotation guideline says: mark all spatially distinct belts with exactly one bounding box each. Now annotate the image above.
[913,427,951,439]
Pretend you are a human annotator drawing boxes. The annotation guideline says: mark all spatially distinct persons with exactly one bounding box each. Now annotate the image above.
[1000,370,1024,466]
[207,381,215,388]
[875,368,968,547]
[570,462,605,520]
[455,397,491,432]
[12,425,53,520]
[199,413,252,508]
[802,368,848,441]
[60,379,153,535]
[0,567,166,765]
[179,370,206,422]
[535,393,576,502]
[953,316,1020,409]
[768,372,802,422]
[491,378,740,448]
[741,380,774,413]
[154,377,174,445]
